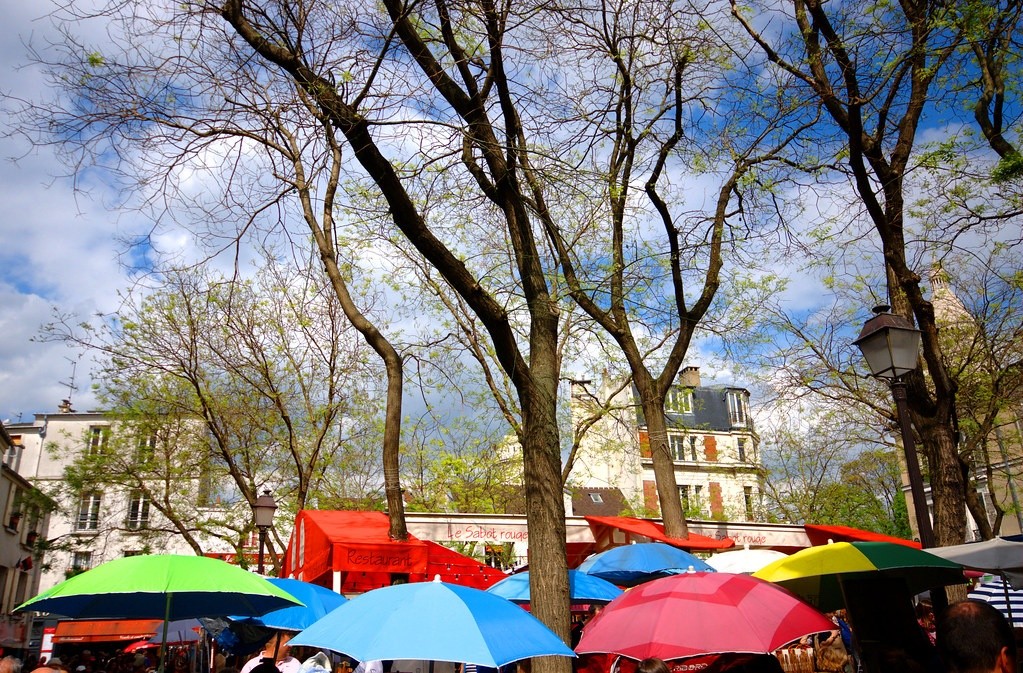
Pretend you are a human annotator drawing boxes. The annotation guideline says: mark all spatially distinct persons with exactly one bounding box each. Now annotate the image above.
[936,597,1017,671]
[0,509,1022,673]
[832,611,859,668]
[799,613,840,671]
[914,597,936,644]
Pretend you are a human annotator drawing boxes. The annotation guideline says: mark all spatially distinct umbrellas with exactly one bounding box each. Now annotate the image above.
[587,541,718,587]
[283,574,577,669]
[223,572,350,665]
[802,524,923,554]
[753,539,968,583]
[573,550,609,573]
[704,548,790,576]
[485,568,625,601]
[574,566,839,662]
[925,533,1022,627]
[9,549,306,673]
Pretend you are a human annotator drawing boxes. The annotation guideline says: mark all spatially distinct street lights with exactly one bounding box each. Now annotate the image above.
[252,488,279,575]
[848,303,949,645]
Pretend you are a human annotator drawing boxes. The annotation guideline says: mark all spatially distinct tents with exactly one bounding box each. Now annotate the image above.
[422,539,511,590]
[584,515,736,551]
[287,508,428,592]
